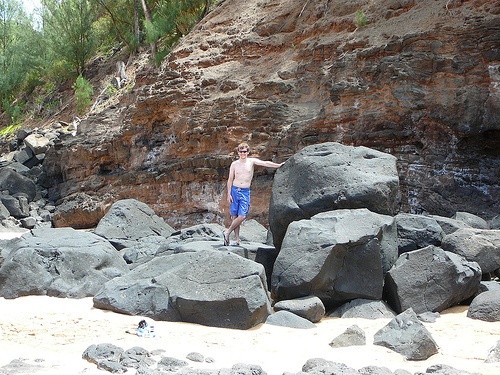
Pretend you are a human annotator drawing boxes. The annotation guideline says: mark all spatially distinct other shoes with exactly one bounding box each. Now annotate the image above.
[223,231,229,246]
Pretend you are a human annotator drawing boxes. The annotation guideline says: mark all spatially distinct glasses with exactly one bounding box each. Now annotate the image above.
[239,151,248,153]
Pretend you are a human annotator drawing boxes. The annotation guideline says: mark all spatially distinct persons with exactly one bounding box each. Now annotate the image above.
[222,143,285,246]
